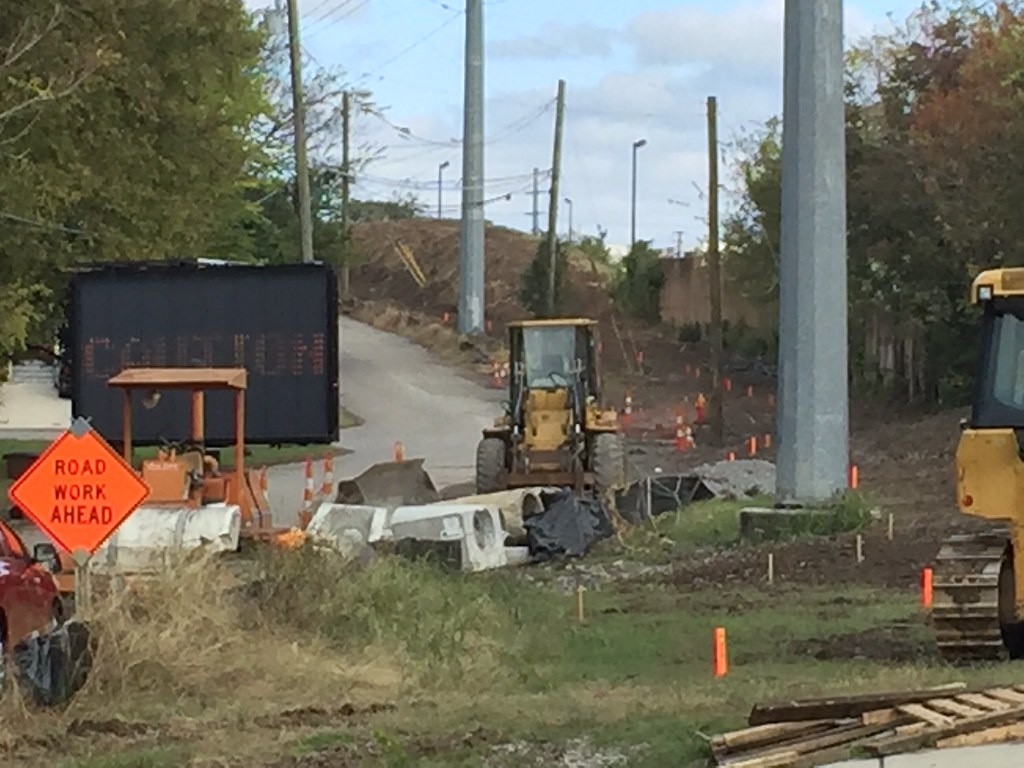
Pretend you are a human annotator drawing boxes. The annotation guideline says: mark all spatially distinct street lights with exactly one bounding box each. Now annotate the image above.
[439,161,449,219]
[632,139,647,244]
[565,198,572,240]
[341,89,371,292]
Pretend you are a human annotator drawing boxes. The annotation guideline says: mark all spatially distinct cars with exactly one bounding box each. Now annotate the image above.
[0,519,63,656]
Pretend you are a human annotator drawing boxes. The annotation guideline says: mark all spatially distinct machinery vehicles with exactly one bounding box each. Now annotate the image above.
[933,267,1024,665]
[52,367,273,595]
[475,319,626,494]
